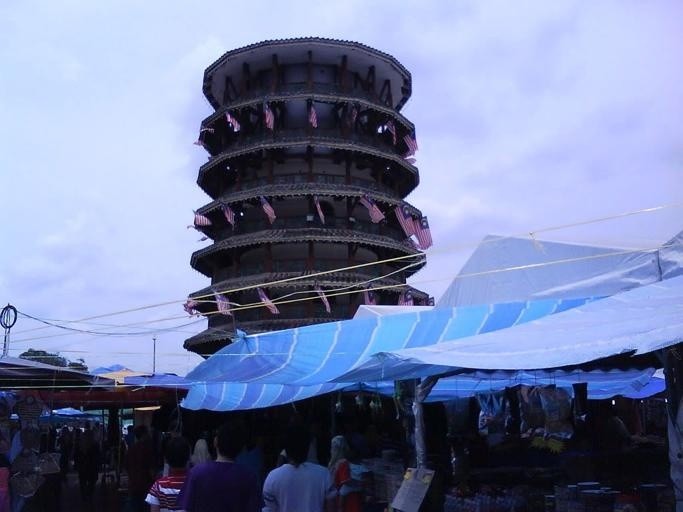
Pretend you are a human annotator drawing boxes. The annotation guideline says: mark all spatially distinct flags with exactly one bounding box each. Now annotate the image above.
[312,195,326,224]
[350,106,358,125]
[395,290,416,307]
[263,103,274,130]
[214,293,233,317]
[359,191,386,224]
[184,296,200,317]
[192,126,212,146]
[419,297,437,307]
[308,102,318,128]
[392,202,415,238]
[412,214,434,250]
[313,284,334,314]
[224,110,240,133]
[402,127,419,157]
[193,212,213,227]
[362,282,378,307]
[256,286,281,315]
[381,117,397,146]
[219,202,235,232]
[259,195,276,225]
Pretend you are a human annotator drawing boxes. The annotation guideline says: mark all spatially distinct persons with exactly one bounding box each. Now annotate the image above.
[0,387,682,511]
